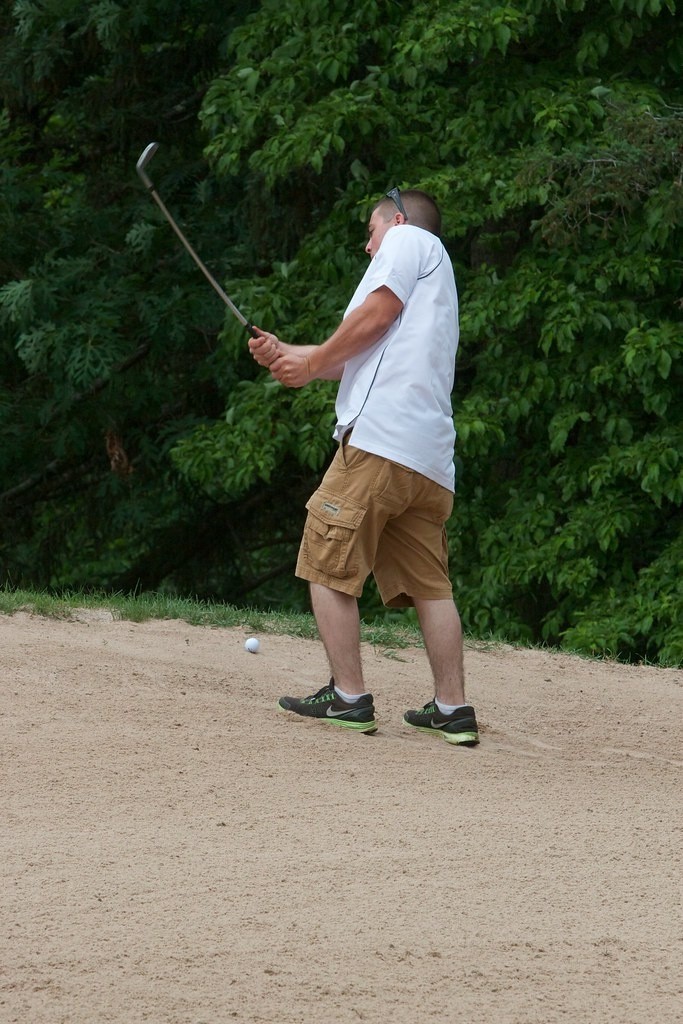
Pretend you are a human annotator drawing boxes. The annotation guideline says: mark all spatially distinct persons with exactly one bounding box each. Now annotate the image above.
[247,188,478,746]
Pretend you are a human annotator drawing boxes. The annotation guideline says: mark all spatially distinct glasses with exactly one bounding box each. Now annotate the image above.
[386,187,408,220]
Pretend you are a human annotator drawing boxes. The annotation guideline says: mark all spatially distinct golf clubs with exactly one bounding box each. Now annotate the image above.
[135,142,260,339]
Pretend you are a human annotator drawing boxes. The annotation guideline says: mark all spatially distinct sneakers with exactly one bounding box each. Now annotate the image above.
[402,697,480,746]
[278,676,378,735]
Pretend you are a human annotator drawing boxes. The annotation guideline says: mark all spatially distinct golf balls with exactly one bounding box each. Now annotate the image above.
[245,637,260,653]
